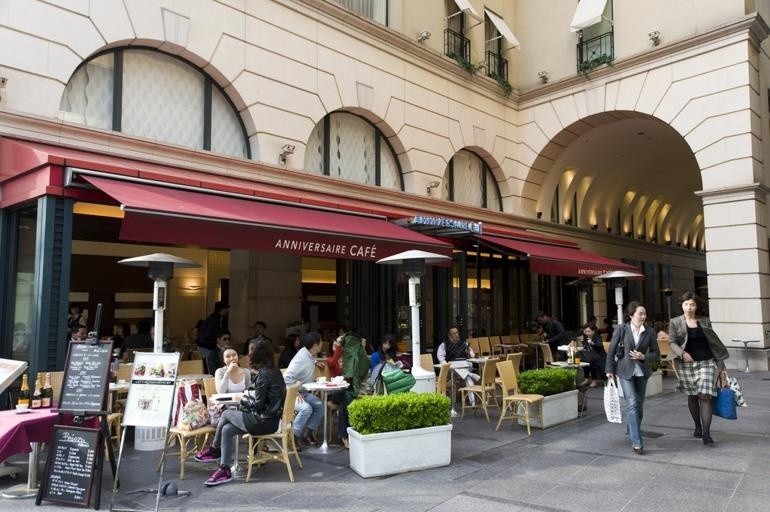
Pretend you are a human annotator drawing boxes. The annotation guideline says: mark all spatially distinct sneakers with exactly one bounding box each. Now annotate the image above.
[192,446,222,463]
[289,429,318,451]
[579,378,604,388]
[693,429,714,446]
[203,464,233,486]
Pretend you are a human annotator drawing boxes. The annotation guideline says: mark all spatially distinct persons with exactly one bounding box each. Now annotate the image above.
[647,318,655,328]
[437,327,481,406]
[196,300,232,375]
[188,320,416,485]
[66,301,176,361]
[666,291,731,443]
[535,310,614,389]
[604,300,660,453]
[655,320,670,339]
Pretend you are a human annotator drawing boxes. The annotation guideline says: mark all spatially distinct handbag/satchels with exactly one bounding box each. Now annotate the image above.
[614,323,626,358]
[711,368,738,420]
[206,403,230,428]
[373,361,388,397]
[603,377,622,424]
[171,377,203,429]
[724,370,748,408]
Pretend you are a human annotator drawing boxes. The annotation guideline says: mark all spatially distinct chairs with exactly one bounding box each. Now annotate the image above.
[362,331,677,421]
[497,359,545,436]
[1,330,350,485]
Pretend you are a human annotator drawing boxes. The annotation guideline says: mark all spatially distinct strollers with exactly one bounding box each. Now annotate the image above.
[355,358,413,399]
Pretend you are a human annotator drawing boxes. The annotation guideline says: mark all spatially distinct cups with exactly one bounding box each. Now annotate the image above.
[16,404,28,410]
[316,375,348,386]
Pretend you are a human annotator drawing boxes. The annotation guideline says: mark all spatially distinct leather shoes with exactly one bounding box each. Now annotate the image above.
[634,447,644,454]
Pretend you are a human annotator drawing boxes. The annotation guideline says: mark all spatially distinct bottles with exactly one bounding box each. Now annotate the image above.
[18,373,53,409]
[567,346,580,365]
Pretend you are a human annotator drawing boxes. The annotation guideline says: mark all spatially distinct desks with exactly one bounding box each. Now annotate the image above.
[732,338,761,374]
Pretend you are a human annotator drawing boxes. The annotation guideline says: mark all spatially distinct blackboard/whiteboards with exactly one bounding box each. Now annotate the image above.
[42,425,100,508]
[56,340,113,414]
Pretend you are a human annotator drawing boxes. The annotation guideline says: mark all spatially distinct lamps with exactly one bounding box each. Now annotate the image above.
[647,30,661,46]
[415,30,431,42]
[277,143,295,166]
[537,70,549,83]
[534,210,672,248]
[426,180,440,194]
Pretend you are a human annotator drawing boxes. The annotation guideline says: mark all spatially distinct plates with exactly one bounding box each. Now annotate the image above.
[14,408,31,413]
